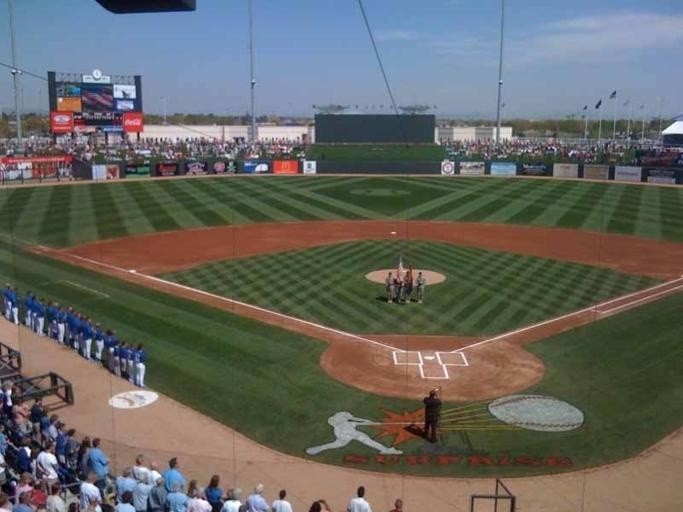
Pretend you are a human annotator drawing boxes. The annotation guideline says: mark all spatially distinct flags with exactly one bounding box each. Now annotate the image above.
[582,90,617,110]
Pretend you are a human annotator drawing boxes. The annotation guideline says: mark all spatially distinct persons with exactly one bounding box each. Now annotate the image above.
[438,134,681,175]
[1,380,225,511]
[413,272,425,304]
[219,482,403,512]
[1,129,304,175]
[2,284,146,387]
[394,272,402,304]
[421,388,441,443]
[402,271,413,304]
[384,272,392,303]
